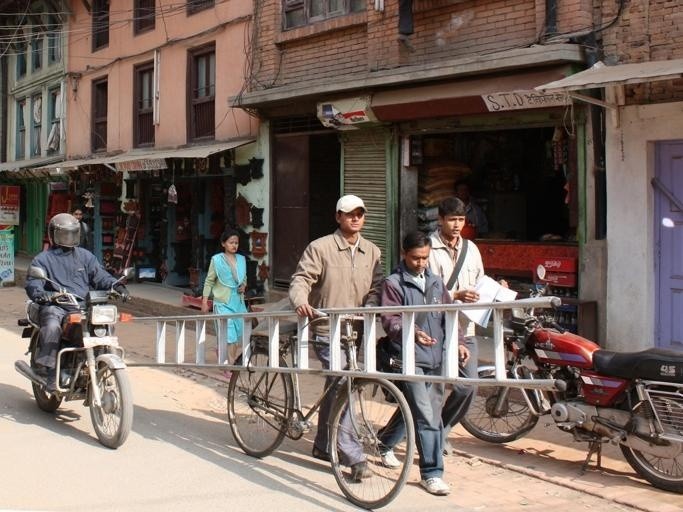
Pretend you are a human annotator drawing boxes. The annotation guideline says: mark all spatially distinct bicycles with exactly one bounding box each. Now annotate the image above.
[227,307,416,510]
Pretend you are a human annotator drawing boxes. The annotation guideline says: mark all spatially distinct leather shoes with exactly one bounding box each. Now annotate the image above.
[314,446,345,462]
[351,464,372,480]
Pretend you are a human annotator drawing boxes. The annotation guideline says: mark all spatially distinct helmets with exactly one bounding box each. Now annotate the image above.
[48,213,80,248]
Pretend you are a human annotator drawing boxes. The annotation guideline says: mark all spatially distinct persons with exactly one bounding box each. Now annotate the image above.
[427,197,508,457]
[371,231,473,497]
[455,181,489,239]
[202,229,246,376]
[72,208,92,250]
[289,194,382,479]
[26,213,129,377]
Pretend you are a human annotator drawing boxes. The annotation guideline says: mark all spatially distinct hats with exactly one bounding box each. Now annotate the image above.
[336,195,368,213]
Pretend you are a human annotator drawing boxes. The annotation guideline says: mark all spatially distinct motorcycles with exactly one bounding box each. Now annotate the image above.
[459,264,682,495]
[15,266,134,449]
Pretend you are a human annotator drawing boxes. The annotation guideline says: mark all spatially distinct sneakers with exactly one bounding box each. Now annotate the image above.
[420,477,450,494]
[47,373,58,391]
[382,449,400,467]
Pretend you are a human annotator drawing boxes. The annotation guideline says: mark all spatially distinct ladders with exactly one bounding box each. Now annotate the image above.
[125,297,564,390]
